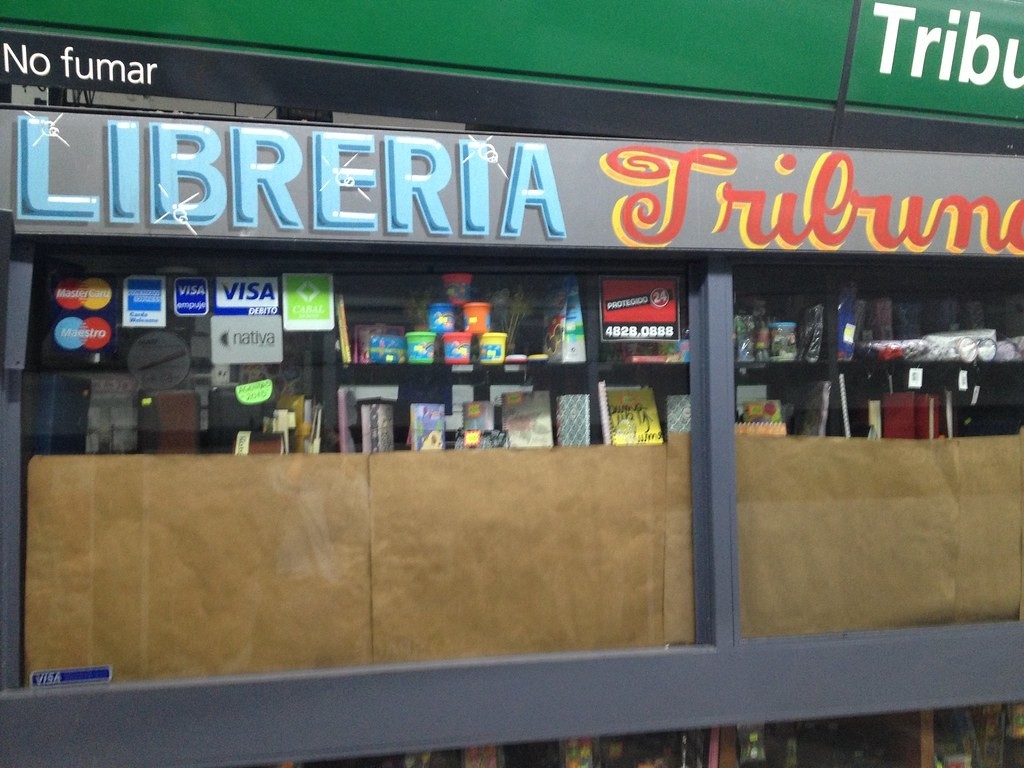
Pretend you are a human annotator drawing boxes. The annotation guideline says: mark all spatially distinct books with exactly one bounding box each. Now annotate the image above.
[735,374,959,442]
[499,390,554,449]
[33,373,323,455]
[361,404,393,454]
[410,403,447,452]
[462,399,494,432]
[554,394,592,447]
[336,386,358,454]
[597,380,664,446]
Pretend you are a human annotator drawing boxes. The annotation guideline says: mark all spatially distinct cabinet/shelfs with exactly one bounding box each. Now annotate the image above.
[318,290,1024,451]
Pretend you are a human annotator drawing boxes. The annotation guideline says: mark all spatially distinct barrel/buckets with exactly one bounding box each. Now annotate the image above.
[442,332,474,364]
[443,273,472,308]
[478,332,507,364]
[426,303,458,335]
[405,331,437,364]
[463,302,492,335]
